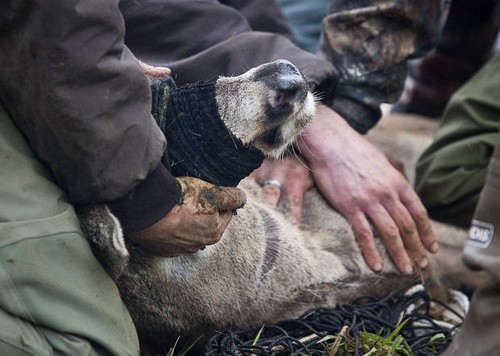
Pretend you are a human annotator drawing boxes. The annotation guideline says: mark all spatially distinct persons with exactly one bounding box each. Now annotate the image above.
[0,0,500,356]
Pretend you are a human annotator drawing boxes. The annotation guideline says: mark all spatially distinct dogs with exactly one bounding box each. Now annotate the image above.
[78,58,486,340]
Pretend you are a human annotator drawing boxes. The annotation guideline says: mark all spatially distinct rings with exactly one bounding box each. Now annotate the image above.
[261,180,283,188]
